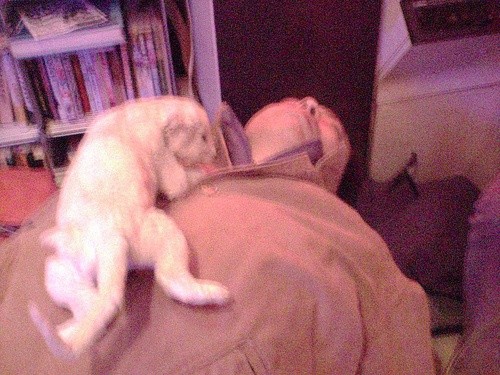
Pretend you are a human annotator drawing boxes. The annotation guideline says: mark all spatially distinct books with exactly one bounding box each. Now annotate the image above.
[2,0,177,174]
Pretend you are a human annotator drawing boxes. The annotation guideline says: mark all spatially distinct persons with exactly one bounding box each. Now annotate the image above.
[0,94,438,375]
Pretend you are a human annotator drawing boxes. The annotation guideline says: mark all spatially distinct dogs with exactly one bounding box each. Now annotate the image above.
[27,95,236,359]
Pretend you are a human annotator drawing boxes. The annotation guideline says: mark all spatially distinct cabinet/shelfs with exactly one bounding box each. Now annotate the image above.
[0,0,223,192]
